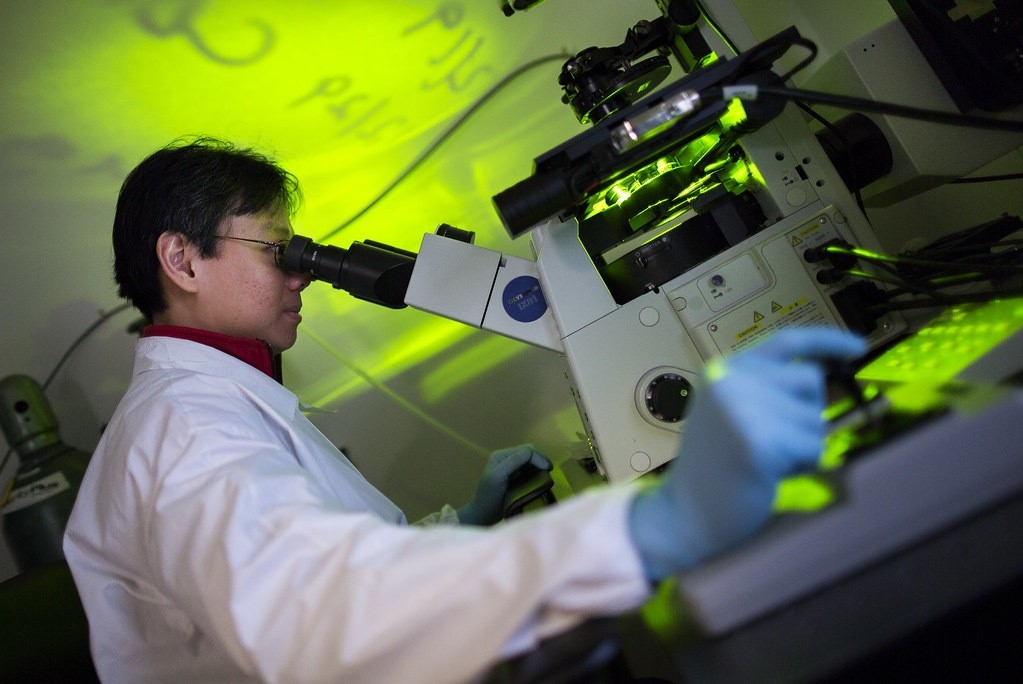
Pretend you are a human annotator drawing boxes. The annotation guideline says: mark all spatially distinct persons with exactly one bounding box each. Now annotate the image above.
[63,133,868,684]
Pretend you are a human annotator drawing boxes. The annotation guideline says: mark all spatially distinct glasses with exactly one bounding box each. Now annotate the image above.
[213,235,290,270]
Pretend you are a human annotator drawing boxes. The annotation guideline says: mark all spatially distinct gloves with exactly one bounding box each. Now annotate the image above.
[626,326,869,588]
[456,444,554,526]
